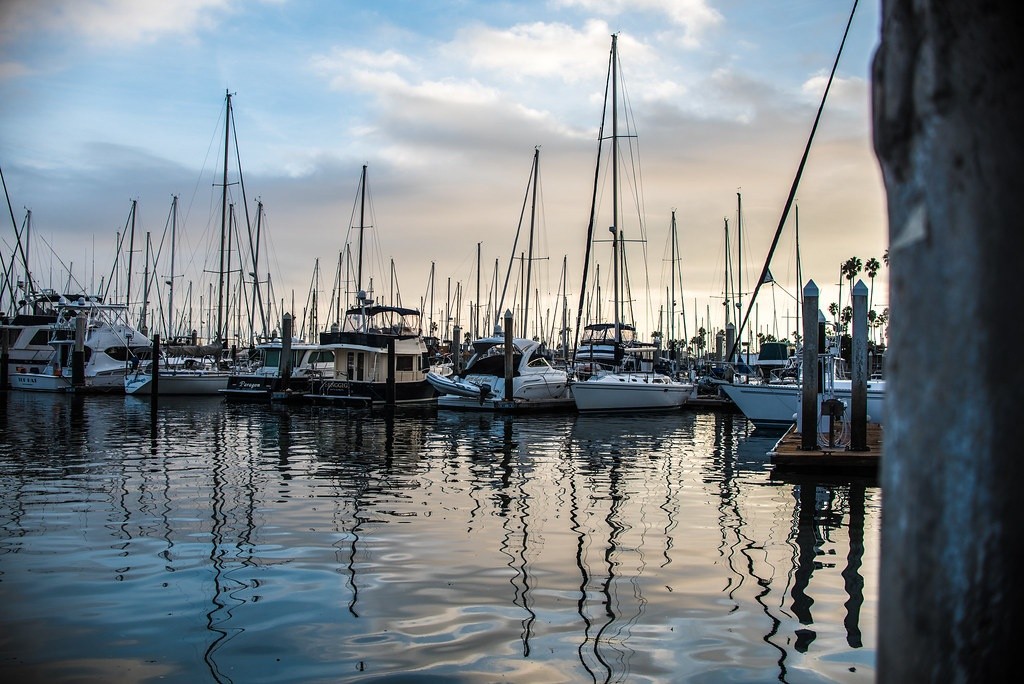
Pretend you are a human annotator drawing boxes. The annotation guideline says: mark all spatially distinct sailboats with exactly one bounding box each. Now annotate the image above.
[1,27,892,433]
